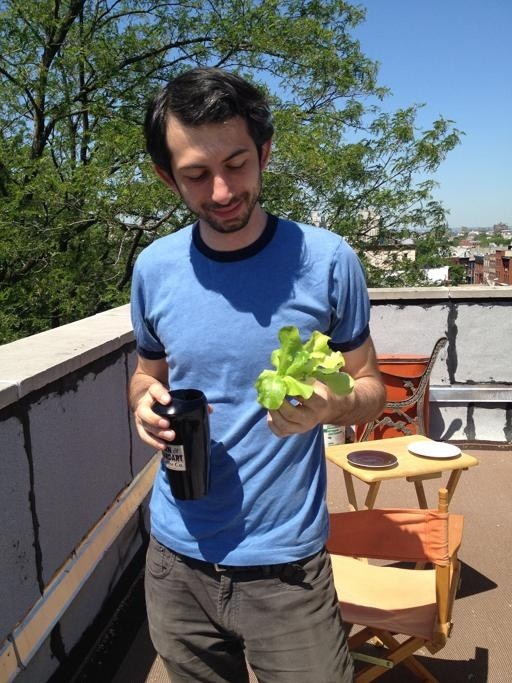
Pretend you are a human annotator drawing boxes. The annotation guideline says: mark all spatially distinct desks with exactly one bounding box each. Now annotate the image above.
[323,433,480,572]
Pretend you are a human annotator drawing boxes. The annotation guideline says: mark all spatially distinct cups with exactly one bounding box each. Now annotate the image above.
[148,388,213,504]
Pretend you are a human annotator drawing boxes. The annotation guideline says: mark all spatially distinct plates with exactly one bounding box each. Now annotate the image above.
[347,449,398,470]
[407,439,464,459]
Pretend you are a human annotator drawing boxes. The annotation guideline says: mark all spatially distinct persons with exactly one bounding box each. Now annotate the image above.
[129,69,388,682]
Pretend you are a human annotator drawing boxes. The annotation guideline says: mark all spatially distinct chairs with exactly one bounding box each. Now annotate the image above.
[318,486,471,682]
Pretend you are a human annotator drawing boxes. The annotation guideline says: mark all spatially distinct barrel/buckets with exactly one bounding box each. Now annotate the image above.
[321,423,346,449]
